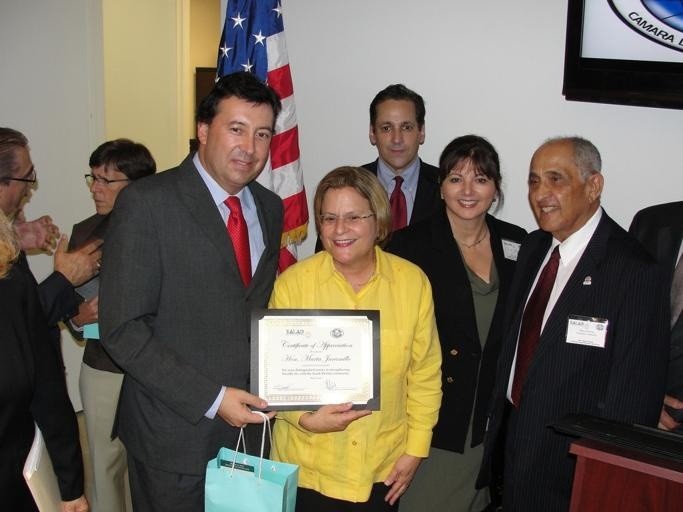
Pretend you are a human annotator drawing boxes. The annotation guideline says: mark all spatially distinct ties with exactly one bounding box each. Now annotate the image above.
[388,177,406,235]
[667,251,683,333]
[224,196,251,287]
[510,245,562,411]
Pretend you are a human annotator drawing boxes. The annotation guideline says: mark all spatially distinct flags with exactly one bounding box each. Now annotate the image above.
[213,0,309,277]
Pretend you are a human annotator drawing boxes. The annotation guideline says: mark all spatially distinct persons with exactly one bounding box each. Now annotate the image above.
[1,123,106,329]
[0,204,91,511]
[96,68,289,511]
[262,165,446,511]
[628,200,683,433]
[62,138,156,511]
[313,82,445,258]
[384,133,529,511]
[472,137,671,511]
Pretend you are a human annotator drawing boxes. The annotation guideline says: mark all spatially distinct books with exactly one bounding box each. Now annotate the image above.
[21,421,64,512]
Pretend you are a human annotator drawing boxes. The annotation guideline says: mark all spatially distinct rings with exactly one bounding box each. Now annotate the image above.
[95,262,101,268]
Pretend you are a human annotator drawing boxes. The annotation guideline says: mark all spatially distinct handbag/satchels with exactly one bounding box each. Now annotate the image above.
[204,409,300,512]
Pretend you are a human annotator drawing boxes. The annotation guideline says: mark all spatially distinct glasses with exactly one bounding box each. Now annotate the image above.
[2,172,36,186]
[79,172,134,188]
